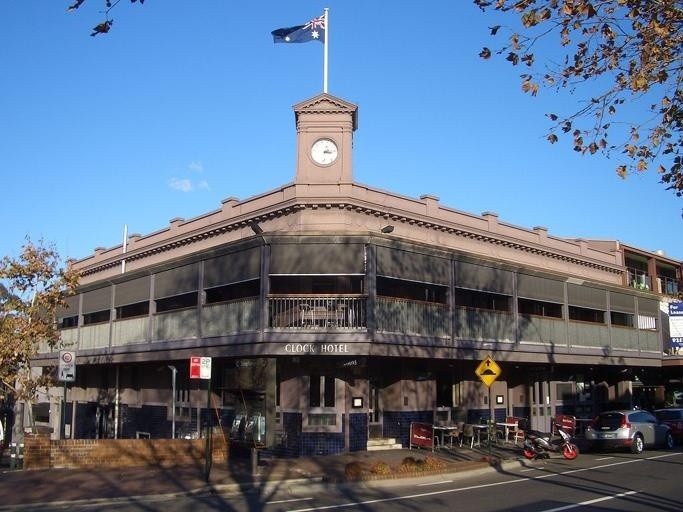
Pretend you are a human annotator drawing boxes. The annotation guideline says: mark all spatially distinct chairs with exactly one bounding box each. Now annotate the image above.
[434,415,528,449]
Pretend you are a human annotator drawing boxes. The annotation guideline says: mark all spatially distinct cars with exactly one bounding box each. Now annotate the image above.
[584,408,674,454]
[652,408,683,446]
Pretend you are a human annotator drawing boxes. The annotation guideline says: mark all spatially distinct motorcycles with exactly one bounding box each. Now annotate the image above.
[517,416,579,460]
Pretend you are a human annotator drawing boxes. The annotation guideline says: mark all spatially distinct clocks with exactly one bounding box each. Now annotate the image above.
[306,135,341,168]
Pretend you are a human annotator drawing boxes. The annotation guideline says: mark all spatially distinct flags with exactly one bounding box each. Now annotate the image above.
[271,14,325,43]
